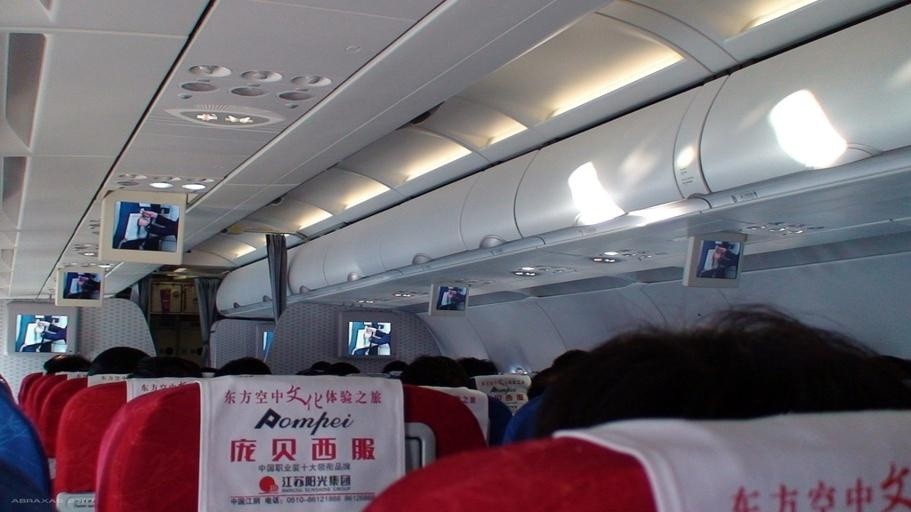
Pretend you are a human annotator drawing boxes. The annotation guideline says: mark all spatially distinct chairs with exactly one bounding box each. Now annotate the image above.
[0,369,911,512]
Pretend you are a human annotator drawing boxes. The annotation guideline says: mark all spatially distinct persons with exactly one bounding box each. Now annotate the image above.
[137,211,178,236]
[77,277,100,290]
[35,321,67,340]
[447,291,466,301]
[364,327,391,344]
[714,247,740,266]
[520,306,911,435]
[42,345,503,387]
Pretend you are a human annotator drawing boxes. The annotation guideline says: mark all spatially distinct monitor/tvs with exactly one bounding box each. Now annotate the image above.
[429,283,470,317]
[337,311,400,361]
[7,303,80,359]
[99,190,187,267]
[56,266,106,308]
[255,322,274,361]
[682,232,748,289]
